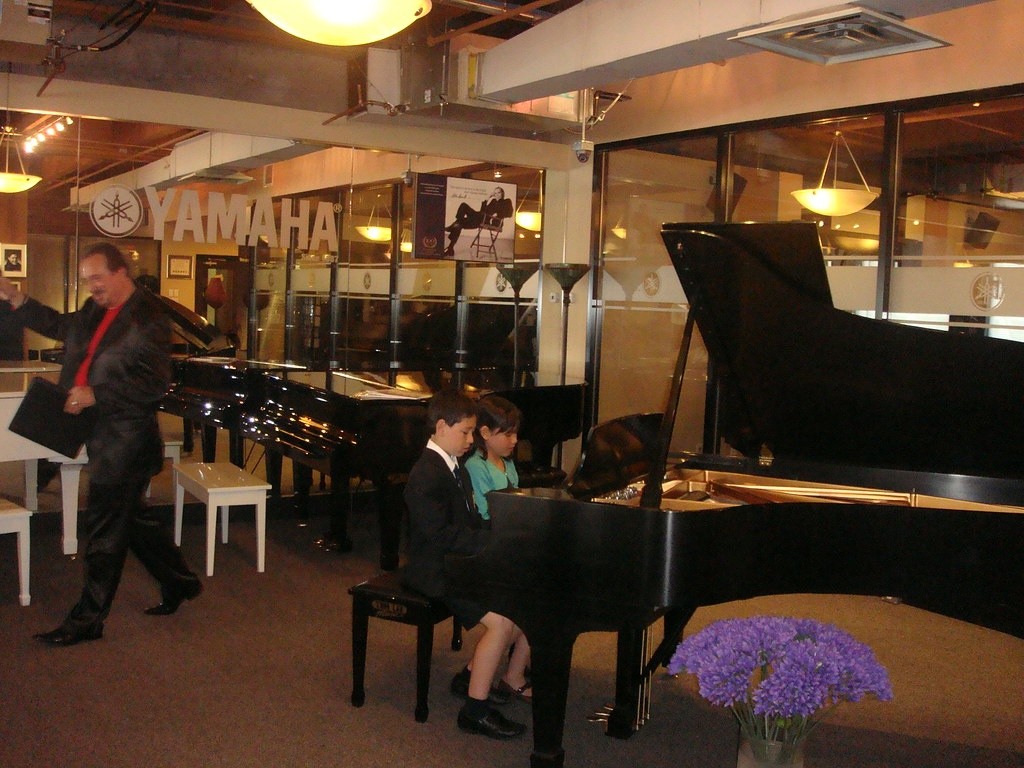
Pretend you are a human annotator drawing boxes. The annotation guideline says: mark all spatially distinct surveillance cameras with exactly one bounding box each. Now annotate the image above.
[401,172,415,188]
[572,142,594,163]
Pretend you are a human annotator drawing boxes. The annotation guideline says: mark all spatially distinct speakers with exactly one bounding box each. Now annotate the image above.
[965,212,1000,249]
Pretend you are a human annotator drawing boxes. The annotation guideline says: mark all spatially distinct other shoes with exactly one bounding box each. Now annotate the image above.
[498,678,534,705]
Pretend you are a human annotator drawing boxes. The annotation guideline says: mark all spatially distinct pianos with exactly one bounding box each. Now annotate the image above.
[0,360,64,512]
[446,222,1024,768]
[43,294,311,468]
[0,372,89,561]
[238,368,587,571]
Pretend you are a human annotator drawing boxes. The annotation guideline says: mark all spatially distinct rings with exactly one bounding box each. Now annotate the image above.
[71,401,79,407]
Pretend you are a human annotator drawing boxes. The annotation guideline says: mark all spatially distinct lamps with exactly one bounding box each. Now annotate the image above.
[246,0,433,47]
[515,169,543,231]
[789,121,882,217]
[0,72,42,193]
[355,194,392,242]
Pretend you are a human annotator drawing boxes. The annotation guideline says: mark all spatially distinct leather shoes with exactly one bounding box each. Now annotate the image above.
[451,673,513,705]
[457,706,525,740]
[144,582,204,615]
[33,624,104,646]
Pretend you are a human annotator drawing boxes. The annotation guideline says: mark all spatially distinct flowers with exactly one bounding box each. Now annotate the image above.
[667,615,892,735]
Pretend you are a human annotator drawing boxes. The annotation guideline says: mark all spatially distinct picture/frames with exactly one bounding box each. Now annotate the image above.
[0,243,27,277]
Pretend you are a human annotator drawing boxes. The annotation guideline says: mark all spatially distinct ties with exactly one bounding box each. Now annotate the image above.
[453,464,470,512]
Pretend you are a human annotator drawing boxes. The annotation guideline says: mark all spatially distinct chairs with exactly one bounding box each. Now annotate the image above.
[470,213,502,260]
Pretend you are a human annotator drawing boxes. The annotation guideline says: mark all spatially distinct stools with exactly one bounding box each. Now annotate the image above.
[347,569,464,722]
[0,498,33,606]
[172,461,272,576]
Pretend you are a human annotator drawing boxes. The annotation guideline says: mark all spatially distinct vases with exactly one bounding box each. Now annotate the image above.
[737,726,804,768]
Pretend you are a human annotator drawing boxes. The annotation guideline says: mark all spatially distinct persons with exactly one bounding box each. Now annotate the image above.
[0,242,204,644]
[5,250,21,271]
[380,389,533,739]
[134,272,172,354]
[445,186,514,257]
[465,394,540,703]
